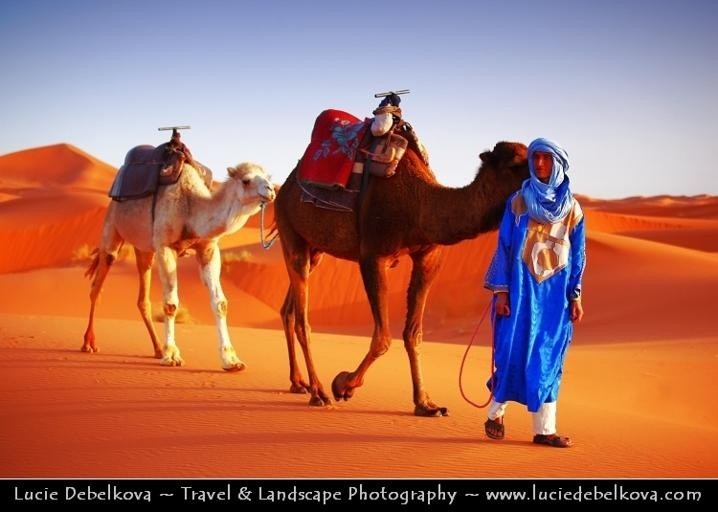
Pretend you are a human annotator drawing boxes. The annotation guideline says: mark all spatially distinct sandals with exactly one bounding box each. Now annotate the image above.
[485,417,504,439]
[534,434,569,447]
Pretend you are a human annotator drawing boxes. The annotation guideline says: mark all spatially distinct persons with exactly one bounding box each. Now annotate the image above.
[483,136,586,449]
